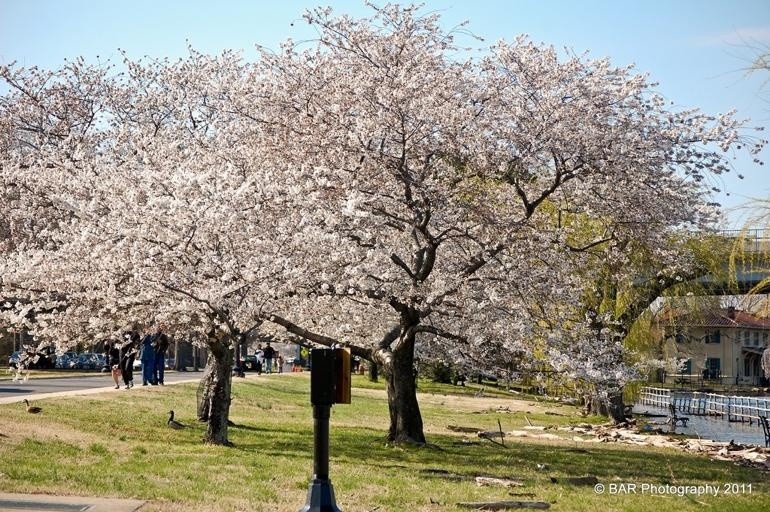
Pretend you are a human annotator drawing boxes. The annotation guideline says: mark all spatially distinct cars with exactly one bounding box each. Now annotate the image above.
[54,352,106,369]
[164,358,175,369]
[472,369,497,381]
[240,355,262,375]
[133,358,142,371]
[8,351,23,368]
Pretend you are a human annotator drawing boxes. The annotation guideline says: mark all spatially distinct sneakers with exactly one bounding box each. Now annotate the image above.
[114,382,133,389]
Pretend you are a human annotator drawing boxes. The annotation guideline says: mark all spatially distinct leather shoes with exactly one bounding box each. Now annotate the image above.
[142,380,164,386]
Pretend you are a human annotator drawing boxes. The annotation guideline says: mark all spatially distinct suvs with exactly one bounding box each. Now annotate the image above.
[24,345,55,369]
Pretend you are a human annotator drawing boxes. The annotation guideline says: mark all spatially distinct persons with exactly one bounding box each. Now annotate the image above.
[103,324,168,389]
[253,342,285,376]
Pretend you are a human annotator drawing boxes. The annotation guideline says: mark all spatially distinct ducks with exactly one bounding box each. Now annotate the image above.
[166,410,187,430]
[22,399,42,414]
